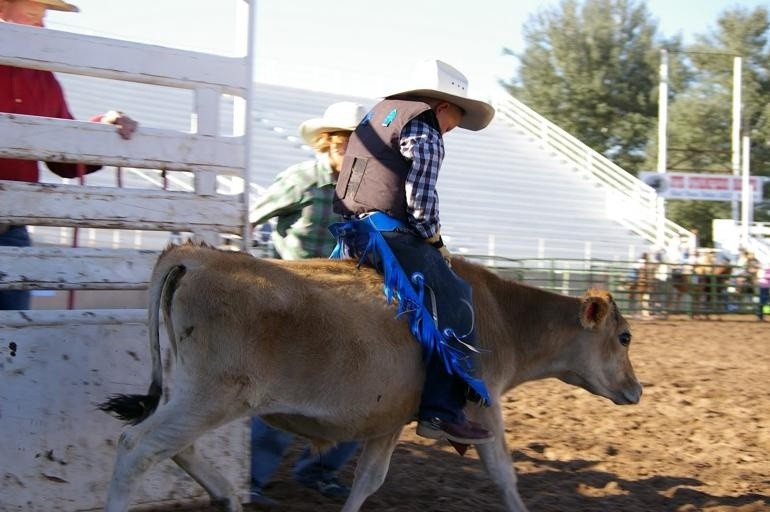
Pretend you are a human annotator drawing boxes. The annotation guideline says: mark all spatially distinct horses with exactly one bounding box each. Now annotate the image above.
[621,260,760,319]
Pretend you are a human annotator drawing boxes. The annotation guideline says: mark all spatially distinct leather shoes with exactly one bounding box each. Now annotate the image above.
[416,415,496,445]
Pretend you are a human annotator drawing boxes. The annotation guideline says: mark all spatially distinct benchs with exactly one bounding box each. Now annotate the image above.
[54,71,691,270]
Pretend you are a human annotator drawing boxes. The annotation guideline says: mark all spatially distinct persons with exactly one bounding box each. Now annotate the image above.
[249,101,375,511]
[0,0,139,310]
[617,244,770,323]
[332,58,498,446]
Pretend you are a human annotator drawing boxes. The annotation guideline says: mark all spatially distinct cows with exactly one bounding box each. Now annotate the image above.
[94,237,644,512]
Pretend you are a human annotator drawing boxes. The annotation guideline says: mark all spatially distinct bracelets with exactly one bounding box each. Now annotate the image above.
[430,234,445,249]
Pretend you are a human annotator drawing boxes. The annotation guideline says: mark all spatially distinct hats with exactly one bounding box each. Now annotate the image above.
[380,58,495,131]
[296,101,367,144]
[33,0,80,12]
